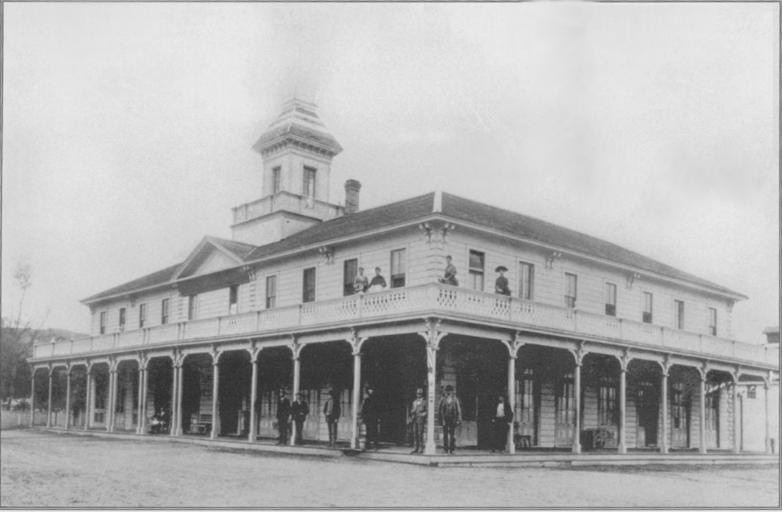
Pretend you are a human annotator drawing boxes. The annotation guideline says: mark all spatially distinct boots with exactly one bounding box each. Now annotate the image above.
[327,438,337,448]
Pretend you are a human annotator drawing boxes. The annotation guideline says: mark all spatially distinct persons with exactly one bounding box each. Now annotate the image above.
[352,266,368,293]
[438,254,459,307]
[291,392,310,445]
[70,400,81,426]
[409,388,427,453]
[493,265,511,315]
[437,385,461,456]
[146,404,171,435]
[361,385,382,449]
[491,393,513,452]
[367,267,386,310]
[274,388,291,446]
[322,390,341,447]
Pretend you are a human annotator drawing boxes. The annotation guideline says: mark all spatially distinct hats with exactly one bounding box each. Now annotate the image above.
[416,388,424,395]
[445,384,455,390]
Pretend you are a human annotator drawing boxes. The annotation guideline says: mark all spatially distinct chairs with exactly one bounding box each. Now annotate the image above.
[514,422,531,450]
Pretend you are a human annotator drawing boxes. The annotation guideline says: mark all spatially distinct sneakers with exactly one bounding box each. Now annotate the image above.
[410,446,424,453]
[275,441,287,446]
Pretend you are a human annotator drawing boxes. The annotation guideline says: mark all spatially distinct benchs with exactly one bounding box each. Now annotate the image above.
[190,414,213,435]
